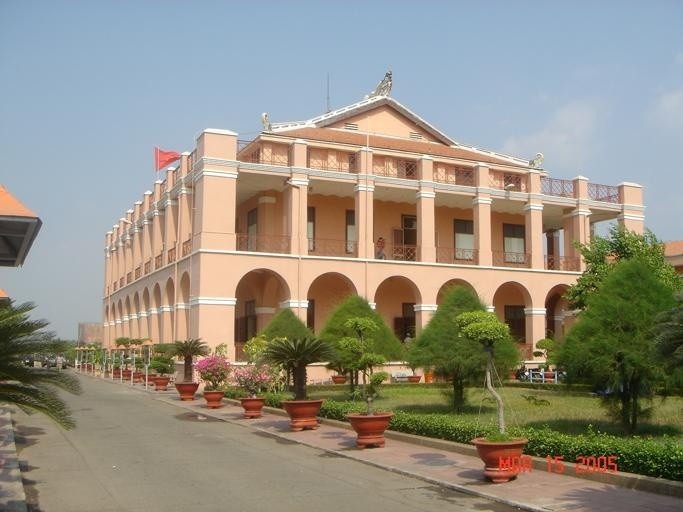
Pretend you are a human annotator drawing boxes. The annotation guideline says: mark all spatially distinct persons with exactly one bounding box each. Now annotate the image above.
[375,237,387,260]
[54,353,66,373]
[403,332,412,343]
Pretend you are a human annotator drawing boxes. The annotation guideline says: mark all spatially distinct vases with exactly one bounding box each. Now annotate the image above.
[239,398,265,420]
[204,391,225,408]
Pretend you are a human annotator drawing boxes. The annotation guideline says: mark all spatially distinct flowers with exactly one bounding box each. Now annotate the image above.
[233,366,270,398]
[194,356,232,392]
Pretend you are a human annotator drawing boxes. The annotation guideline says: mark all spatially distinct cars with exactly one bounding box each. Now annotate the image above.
[41,357,49,367]
[20,356,34,367]
[46,356,66,369]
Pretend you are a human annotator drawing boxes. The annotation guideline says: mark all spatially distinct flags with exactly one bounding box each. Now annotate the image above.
[154,145,181,172]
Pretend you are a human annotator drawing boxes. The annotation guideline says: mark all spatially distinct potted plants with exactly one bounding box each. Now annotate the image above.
[268,315,422,448]
[111,338,211,401]
[457,311,555,484]
